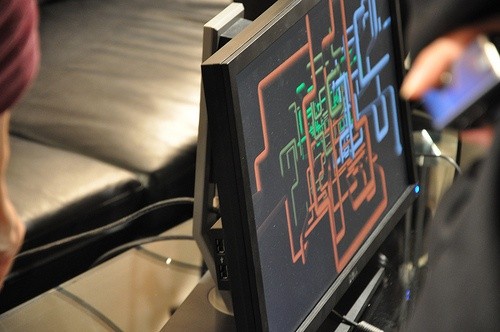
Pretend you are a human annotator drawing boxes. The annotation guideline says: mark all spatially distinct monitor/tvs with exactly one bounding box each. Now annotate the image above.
[201,0,421,332]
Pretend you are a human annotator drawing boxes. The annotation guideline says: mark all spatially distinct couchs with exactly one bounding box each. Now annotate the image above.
[2,2,229,316]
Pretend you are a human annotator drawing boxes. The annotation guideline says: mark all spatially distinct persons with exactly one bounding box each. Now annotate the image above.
[1,0,42,293]
